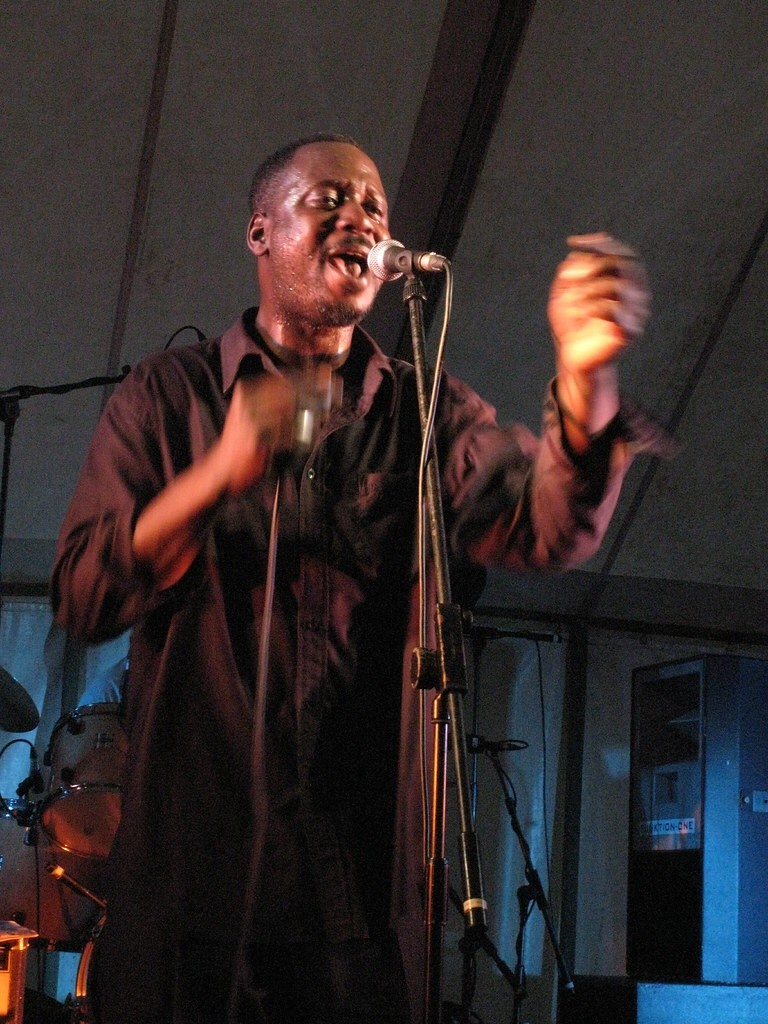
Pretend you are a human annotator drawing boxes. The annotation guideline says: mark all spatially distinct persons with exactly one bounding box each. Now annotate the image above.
[54,138,651,1024]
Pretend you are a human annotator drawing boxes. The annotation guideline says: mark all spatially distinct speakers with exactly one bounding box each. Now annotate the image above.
[624,653,768,987]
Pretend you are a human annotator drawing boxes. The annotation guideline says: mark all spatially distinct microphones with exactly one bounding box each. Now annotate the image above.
[368,238,451,280]
[28,747,46,793]
[466,734,528,756]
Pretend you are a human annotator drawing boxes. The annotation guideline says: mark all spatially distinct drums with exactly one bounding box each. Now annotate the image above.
[0,797,112,953]
[40,702,133,858]
